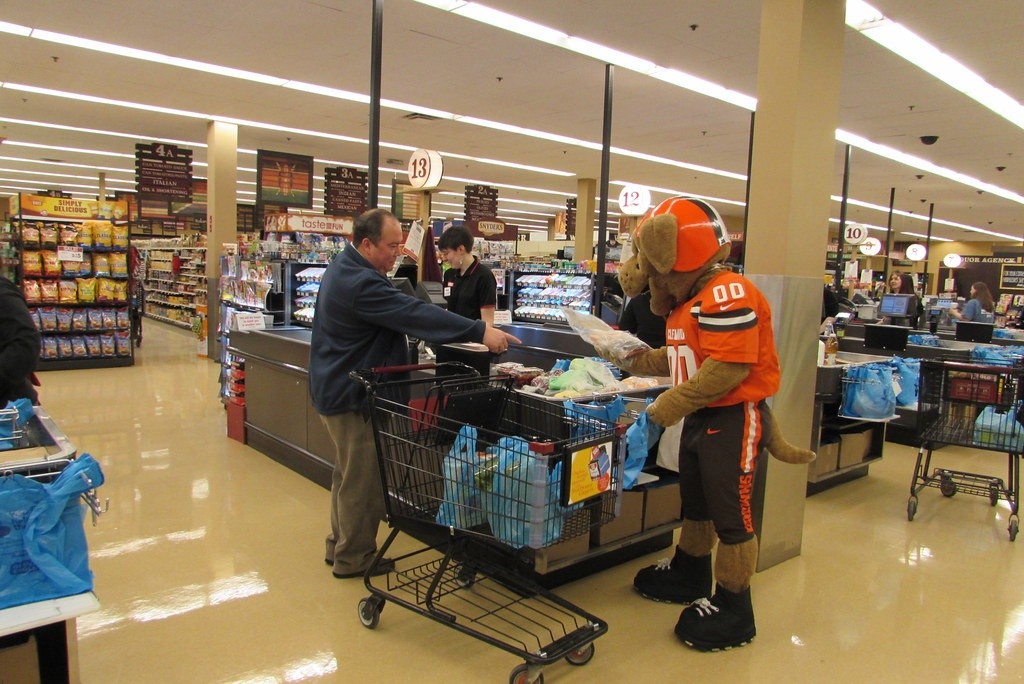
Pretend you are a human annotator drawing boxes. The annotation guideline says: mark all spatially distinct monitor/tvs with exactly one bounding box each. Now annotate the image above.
[880,293,910,318]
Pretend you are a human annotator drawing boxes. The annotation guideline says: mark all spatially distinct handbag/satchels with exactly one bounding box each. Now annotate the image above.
[435,426,587,547]
[972,400,1024,452]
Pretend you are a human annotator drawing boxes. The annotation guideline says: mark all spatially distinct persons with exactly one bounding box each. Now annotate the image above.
[820,287,840,335]
[0,275,42,409]
[882,272,923,327]
[437,226,499,453]
[130,245,141,340]
[949,282,995,323]
[308,208,522,578]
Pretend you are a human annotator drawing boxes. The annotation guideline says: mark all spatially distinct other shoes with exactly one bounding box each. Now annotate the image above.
[333,557,396,578]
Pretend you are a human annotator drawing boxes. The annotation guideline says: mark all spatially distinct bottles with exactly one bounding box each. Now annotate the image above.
[823,319,832,336]
[824,333,838,366]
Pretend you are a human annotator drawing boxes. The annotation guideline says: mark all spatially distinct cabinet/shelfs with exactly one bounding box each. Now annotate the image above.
[0,213,594,409]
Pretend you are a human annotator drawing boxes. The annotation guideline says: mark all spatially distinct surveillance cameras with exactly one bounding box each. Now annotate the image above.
[916,175,924,180]
[920,136,939,144]
[996,166,1006,171]
[920,200,925,202]
[976,191,983,194]
[988,222,993,224]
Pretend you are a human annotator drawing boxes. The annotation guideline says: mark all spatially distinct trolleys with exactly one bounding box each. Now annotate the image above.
[346,360,627,684]
[132,278,145,347]
[907,357,1024,543]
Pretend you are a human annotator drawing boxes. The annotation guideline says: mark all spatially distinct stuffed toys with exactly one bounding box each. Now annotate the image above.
[594,196,816,651]
[615,256,667,350]
